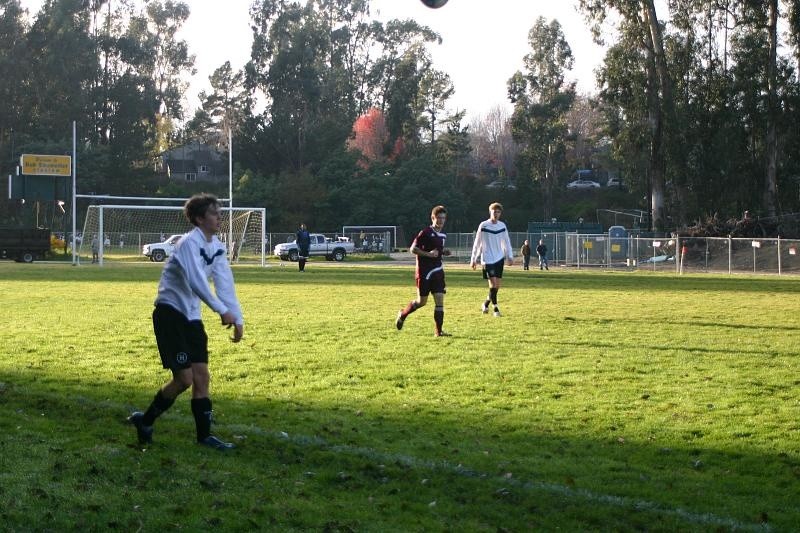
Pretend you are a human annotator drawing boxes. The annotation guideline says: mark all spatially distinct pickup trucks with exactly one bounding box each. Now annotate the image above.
[273,234,355,262]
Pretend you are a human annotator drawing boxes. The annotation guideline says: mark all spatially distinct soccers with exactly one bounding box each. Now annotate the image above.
[419,0,449,9]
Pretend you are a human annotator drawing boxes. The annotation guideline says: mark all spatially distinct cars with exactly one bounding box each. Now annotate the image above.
[485,180,517,190]
[566,180,601,189]
[142,235,184,262]
[607,178,625,188]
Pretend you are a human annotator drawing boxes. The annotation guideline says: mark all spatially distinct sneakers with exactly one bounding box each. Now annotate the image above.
[396,309,405,330]
[482,302,489,313]
[493,305,500,317]
[127,412,154,447]
[196,436,236,449]
[435,331,453,337]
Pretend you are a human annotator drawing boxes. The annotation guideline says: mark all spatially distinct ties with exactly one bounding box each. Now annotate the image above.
[200,247,224,265]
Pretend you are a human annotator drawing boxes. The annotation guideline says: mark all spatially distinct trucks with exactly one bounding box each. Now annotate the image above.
[0,228,51,263]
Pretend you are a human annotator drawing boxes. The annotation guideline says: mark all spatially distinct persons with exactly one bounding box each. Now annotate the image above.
[91,234,99,264]
[296,223,310,272]
[471,202,514,319]
[131,194,246,449]
[537,239,549,270]
[56,230,384,261]
[521,240,531,270]
[396,205,452,337]
